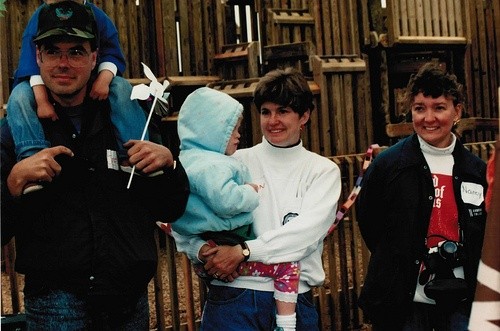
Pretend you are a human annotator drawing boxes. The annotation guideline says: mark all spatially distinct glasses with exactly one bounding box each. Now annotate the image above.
[40,48,91,66]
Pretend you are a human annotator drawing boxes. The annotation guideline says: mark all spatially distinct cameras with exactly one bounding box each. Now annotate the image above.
[425,239,470,274]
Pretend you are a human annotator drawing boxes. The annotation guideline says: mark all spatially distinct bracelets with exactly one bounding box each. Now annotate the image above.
[200,256,207,262]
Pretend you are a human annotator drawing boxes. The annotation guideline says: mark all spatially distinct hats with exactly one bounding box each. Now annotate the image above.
[32,2,99,49]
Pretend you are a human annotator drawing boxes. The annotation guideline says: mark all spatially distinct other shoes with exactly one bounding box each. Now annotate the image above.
[24,182,44,195]
[119,158,164,178]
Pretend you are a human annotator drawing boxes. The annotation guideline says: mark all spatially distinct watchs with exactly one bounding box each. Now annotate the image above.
[239,240,250,263]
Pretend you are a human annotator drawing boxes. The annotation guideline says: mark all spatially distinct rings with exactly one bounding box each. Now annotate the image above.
[214,273,218,278]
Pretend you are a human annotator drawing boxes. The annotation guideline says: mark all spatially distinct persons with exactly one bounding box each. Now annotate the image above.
[173,68,341,331]
[355,64,489,331]
[6,0,164,195]
[169,87,300,331]
[0,1,190,331]
